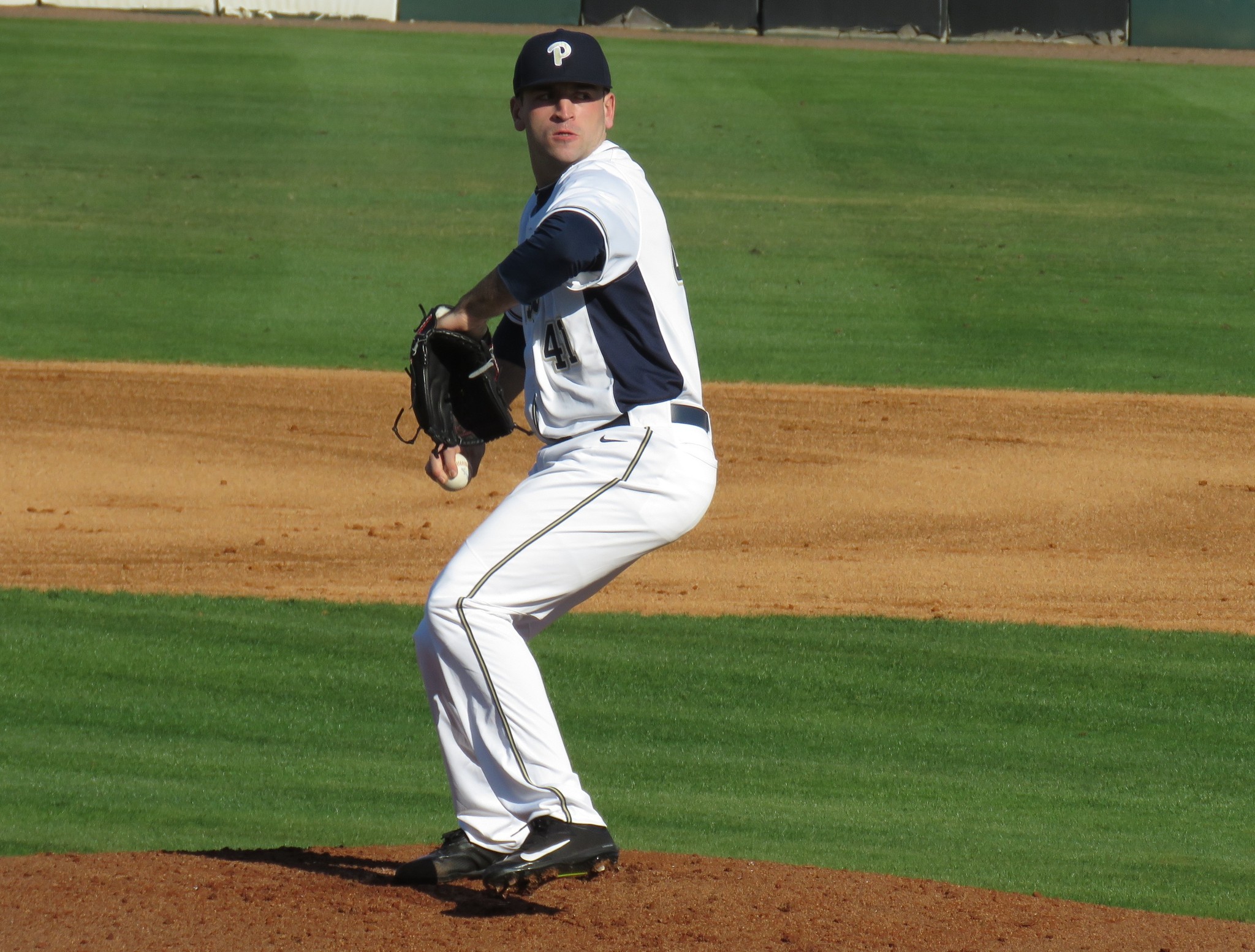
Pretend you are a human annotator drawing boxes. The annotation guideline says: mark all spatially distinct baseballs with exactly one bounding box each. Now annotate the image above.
[437,454,475,491]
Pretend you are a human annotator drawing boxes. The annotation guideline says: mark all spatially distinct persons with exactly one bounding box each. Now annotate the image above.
[393,29,718,896]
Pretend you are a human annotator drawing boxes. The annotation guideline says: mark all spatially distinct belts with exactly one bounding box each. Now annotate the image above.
[560,403,709,441]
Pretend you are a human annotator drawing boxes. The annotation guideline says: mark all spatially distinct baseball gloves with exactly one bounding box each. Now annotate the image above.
[409,305,516,447]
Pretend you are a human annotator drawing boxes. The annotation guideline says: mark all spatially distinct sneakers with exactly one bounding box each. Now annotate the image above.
[396,830,508,884]
[484,816,618,894]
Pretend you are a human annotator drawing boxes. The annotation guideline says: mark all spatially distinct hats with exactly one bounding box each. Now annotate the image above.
[513,29,613,96]
